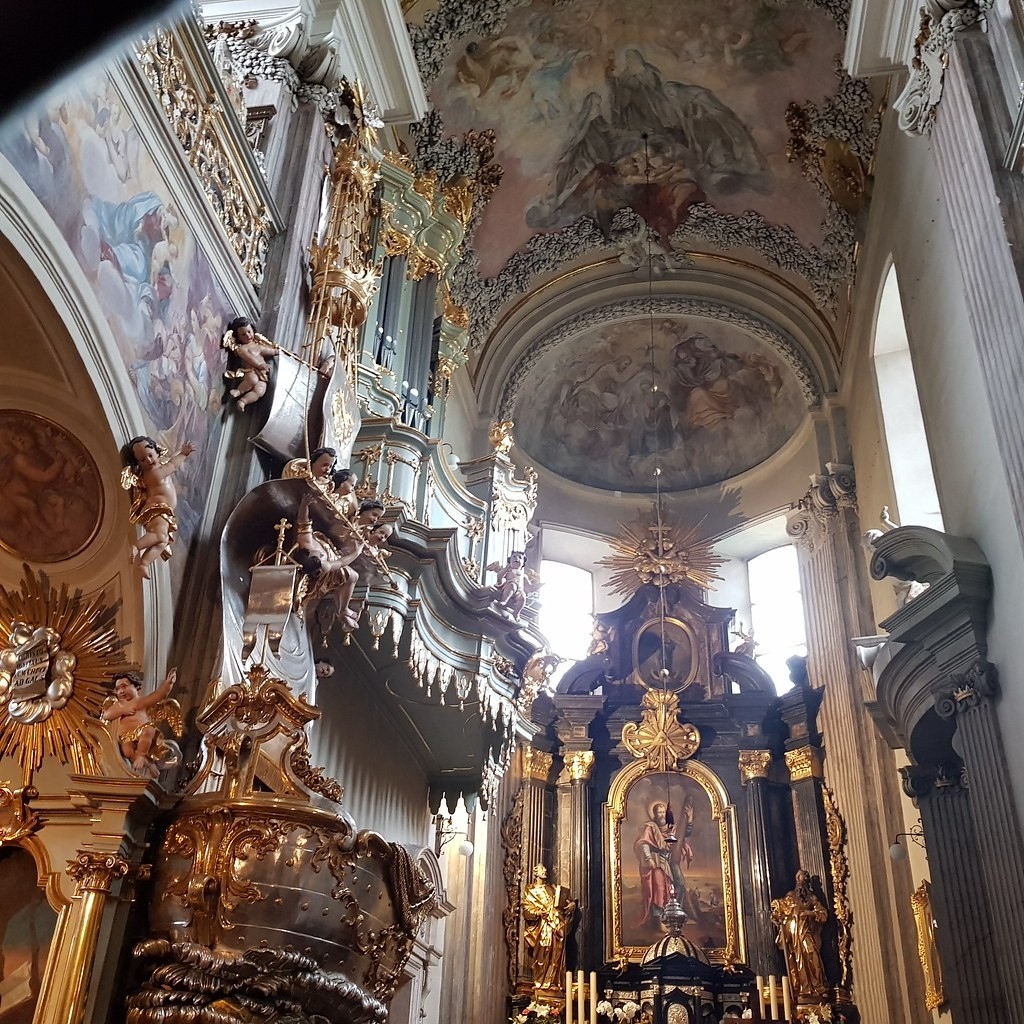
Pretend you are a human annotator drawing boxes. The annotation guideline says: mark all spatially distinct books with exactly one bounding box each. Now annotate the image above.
[554,885,570,908]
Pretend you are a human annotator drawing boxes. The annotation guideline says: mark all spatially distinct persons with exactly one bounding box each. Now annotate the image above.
[123,436,197,580]
[860,505,922,608]
[314,658,335,707]
[296,448,393,629]
[523,864,577,989]
[771,870,828,994]
[104,666,177,777]
[499,551,536,620]
[735,622,759,659]
[229,317,279,412]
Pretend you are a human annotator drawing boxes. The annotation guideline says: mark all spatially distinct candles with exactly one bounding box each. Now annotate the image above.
[781,975,792,1021]
[768,973,779,1021]
[589,970,598,1024]
[576,968,585,1024]
[565,970,573,1024]
[756,972,767,1020]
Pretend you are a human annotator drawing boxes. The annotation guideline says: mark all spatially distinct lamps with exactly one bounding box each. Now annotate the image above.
[429,812,479,861]
[886,813,931,871]
